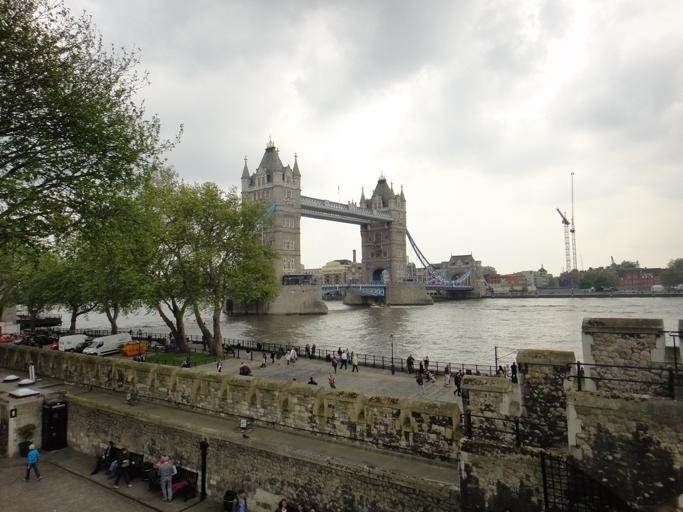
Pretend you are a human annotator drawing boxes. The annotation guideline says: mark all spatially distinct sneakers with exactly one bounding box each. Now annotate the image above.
[36,475,42,481]
[113,485,119,489]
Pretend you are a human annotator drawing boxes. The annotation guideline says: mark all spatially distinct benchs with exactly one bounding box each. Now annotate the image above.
[104,447,198,501]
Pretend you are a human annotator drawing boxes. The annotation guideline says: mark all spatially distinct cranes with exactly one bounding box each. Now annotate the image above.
[556,172,577,270]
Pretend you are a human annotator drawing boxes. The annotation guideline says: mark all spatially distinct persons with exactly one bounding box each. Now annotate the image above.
[22,444,43,481]
[231,490,248,512]
[297,503,305,512]
[89,440,114,475]
[580,367,584,377]
[147,455,166,491]
[309,506,316,512]
[112,447,136,489]
[107,460,118,478]
[274,498,289,512]
[158,456,178,503]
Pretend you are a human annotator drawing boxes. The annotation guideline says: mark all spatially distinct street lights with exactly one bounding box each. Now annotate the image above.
[388,334,396,375]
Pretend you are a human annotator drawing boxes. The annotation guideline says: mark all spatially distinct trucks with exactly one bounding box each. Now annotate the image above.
[58,332,133,356]
